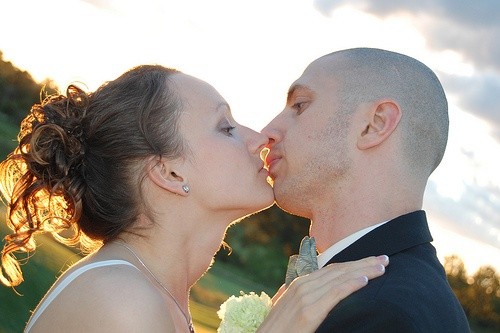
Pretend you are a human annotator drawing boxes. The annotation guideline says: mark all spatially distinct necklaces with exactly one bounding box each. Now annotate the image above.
[104,240,195,333]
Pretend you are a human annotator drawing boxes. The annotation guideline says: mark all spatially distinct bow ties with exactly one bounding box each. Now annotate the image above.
[285,236,319,288]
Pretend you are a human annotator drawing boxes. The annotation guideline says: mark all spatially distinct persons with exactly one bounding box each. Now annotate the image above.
[0,65,275,333]
[261,48,471,333]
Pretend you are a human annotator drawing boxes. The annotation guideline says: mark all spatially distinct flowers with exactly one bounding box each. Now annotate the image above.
[216,291,272,333]
